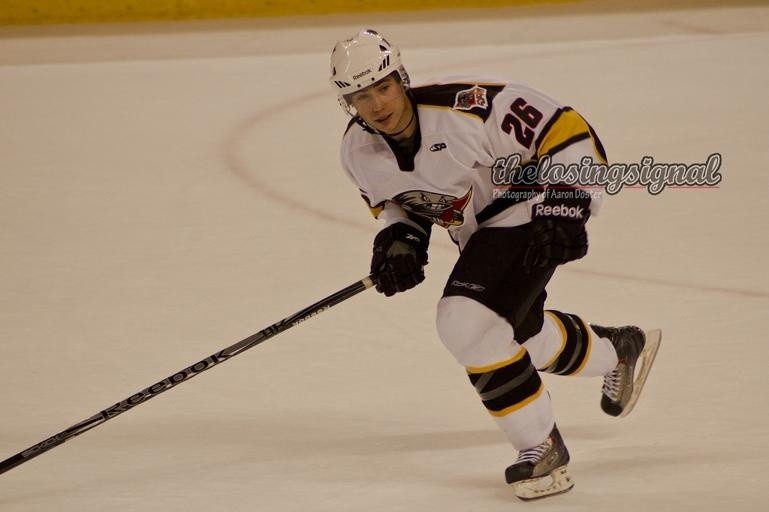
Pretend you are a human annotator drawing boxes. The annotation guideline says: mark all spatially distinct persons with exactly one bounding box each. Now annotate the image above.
[328,27,647,487]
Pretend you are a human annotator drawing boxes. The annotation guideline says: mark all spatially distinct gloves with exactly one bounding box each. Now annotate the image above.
[370,221,430,296]
[531,184,591,274]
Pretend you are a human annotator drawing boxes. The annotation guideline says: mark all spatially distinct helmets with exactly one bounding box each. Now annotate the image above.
[329,30,410,114]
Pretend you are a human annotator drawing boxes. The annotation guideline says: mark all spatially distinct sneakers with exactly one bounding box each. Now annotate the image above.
[505,423,570,484]
[590,325,644,416]
